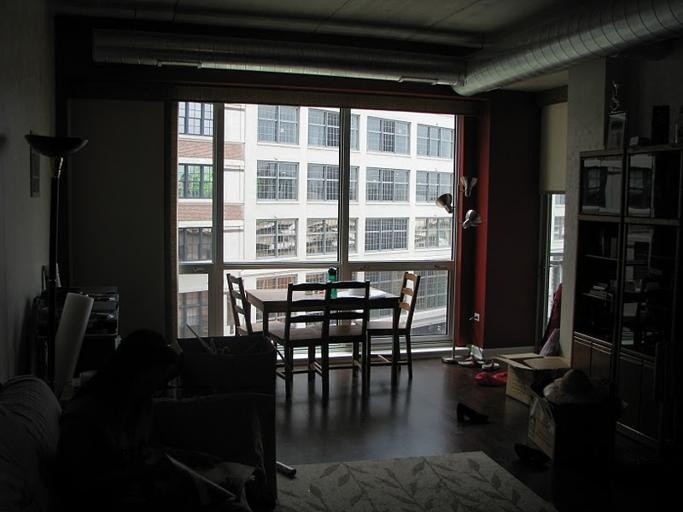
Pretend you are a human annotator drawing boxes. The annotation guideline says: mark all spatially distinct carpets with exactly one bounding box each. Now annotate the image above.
[276,451,561,512]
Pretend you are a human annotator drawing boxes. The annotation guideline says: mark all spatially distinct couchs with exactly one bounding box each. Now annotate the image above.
[0,375,276,512]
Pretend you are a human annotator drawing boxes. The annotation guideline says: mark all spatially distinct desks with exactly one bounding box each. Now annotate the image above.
[245,287,401,399]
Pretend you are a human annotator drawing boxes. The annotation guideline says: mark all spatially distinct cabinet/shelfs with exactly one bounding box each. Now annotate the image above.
[572,147,683,471]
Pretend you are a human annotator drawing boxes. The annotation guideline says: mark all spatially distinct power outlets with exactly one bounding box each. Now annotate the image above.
[473,313,480,322]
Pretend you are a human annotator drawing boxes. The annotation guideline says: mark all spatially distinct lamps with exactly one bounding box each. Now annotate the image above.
[24,133,88,390]
[436,176,483,365]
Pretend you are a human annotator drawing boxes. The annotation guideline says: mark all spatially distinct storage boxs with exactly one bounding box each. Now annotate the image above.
[491,351,569,407]
[527,382,628,463]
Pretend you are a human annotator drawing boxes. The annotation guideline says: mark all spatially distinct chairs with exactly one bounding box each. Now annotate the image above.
[227,273,293,337]
[268,283,329,405]
[352,272,421,379]
[314,281,371,405]
